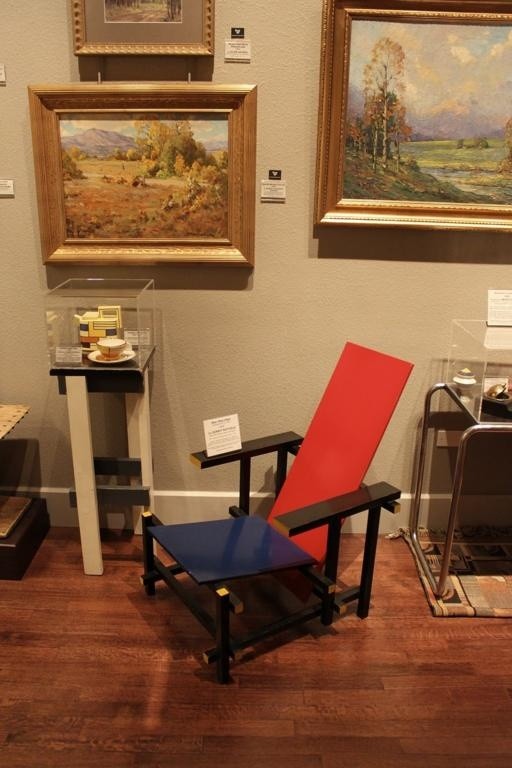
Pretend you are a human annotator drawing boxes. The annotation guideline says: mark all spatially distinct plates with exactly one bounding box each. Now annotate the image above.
[89,348,136,365]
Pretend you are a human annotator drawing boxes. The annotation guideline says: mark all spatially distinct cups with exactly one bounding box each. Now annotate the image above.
[95,339,126,359]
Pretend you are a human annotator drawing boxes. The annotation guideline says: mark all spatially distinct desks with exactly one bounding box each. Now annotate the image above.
[409,380,512,597]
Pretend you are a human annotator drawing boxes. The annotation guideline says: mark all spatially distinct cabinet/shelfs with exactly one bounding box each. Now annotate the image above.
[48,343,158,576]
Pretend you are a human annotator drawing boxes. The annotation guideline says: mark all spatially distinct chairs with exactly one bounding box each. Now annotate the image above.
[140,339,415,686]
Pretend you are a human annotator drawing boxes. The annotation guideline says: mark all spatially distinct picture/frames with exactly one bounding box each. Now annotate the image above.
[27,80,259,270]
[68,0,217,61]
[311,0,512,238]
